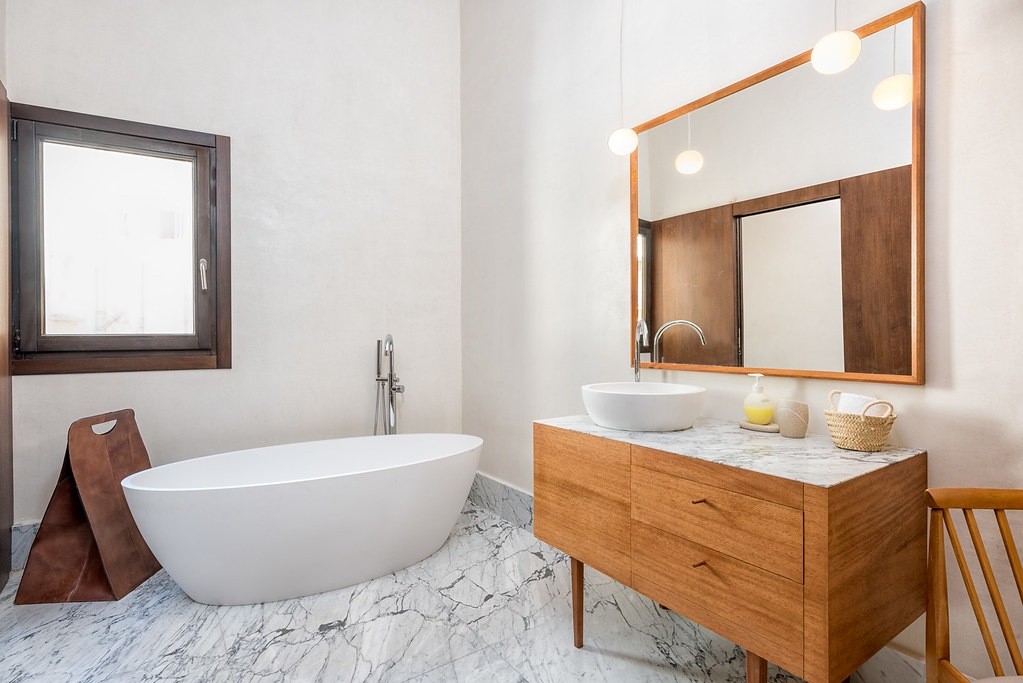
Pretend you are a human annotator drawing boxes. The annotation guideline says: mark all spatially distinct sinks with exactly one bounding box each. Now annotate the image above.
[581,382,707,432]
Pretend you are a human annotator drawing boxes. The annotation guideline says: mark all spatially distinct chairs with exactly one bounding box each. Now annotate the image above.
[924,487,1023,683]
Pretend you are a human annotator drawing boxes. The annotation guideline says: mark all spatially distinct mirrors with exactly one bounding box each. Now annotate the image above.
[628,0,926,385]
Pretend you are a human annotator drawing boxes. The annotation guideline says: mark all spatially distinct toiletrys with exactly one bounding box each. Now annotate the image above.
[744,373,774,425]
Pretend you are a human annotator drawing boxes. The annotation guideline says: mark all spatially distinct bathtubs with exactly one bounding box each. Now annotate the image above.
[120,433,484,606]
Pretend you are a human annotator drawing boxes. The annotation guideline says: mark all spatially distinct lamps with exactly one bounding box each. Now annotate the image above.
[810,0,862,75]
[608,2,638,154]
[676,112,703,176]
[873,26,913,112]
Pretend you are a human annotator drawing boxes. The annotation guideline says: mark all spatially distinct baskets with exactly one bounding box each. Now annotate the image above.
[825,391,897,452]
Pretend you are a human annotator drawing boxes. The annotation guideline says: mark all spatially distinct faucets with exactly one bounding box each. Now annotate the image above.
[653,320,706,363]
[384,334,405,395]
[635,319,650,382]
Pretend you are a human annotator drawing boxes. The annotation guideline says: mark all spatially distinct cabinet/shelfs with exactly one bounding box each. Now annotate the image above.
[534,414,931,683]
